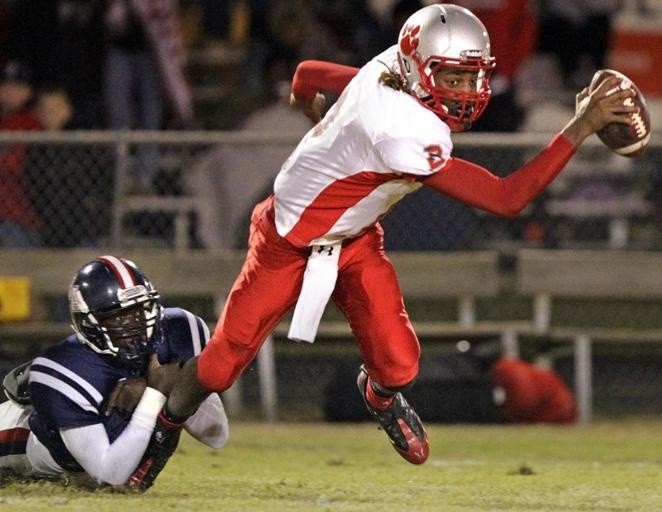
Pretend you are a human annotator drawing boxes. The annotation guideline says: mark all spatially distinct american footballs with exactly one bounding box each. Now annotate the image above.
[589,68,651,157]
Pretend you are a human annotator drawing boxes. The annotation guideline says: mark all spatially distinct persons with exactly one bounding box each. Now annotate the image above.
[115,3,638,497]
[1,259,231,493]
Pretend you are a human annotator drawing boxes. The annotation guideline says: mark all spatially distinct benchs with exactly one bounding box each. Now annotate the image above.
[0,248,242,422]
[236,245,524,426]
[515,245,660,420]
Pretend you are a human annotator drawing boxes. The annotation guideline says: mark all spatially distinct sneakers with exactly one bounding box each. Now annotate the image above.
[124,414,182,494]
[356,363,429,464]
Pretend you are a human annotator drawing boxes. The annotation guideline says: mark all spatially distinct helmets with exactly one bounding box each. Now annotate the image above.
[396,3,497,120]
[68,253,158,356]
